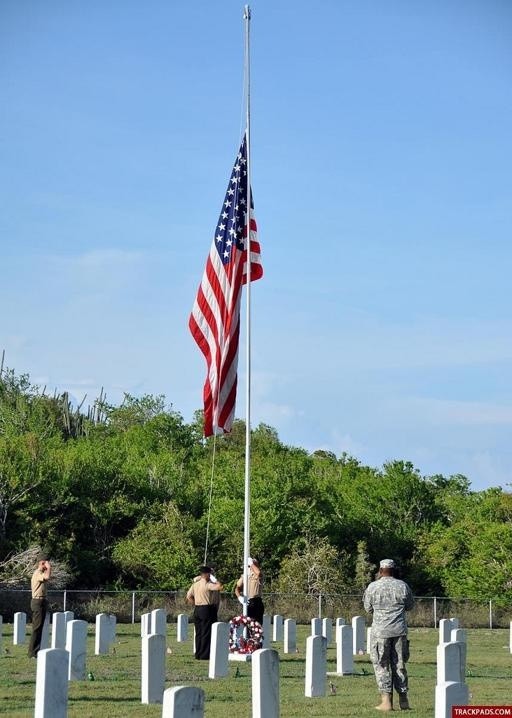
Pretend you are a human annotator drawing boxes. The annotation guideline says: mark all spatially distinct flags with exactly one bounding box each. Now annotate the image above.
[187,127,263,441]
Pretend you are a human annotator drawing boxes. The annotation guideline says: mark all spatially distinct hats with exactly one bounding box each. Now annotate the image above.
[38,554,50,560]
[380,559,394,568]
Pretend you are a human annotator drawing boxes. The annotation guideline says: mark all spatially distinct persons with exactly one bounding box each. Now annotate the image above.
[187,566,224,660]
[363,558,416,713]
[234,558,265,627]
[193,568,220,622]
[27,553,52,659]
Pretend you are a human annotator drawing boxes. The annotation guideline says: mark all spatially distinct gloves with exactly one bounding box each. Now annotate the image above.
[238,596,247,604]
[210,575,217,583]
[248,557,253,565]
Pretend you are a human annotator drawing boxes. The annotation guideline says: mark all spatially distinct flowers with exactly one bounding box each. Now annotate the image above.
[229,616,263,654]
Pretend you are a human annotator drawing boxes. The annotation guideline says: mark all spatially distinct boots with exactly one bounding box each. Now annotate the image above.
[398,693,409,710]
[375,692,394,710]
